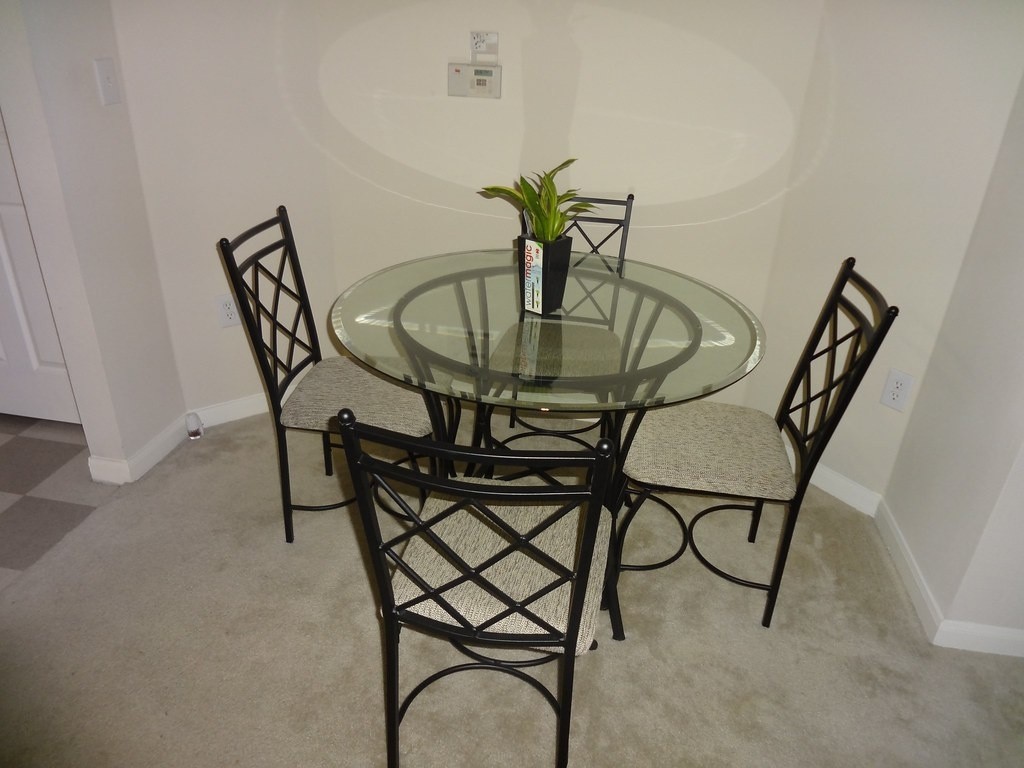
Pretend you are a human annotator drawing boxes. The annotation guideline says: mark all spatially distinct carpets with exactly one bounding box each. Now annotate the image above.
[0,414,116,590]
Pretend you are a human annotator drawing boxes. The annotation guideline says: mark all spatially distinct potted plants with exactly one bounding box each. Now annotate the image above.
[482,158,601,314]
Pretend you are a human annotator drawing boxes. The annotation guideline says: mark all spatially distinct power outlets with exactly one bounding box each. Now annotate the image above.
[880,368,914,412]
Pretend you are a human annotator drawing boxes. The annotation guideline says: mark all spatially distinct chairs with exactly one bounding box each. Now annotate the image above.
[217,205,448,543]
[589,256,901,630]
[461,191,635,477]
[338,409,610,767]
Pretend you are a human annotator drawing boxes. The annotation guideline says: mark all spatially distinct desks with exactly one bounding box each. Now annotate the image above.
[328,246,769,642]
